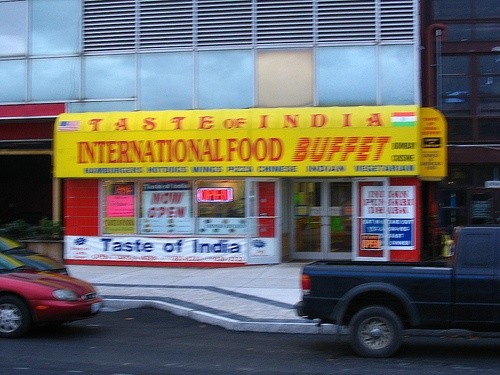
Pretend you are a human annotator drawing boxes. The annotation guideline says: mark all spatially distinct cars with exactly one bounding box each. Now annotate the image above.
[0,253,102,334]
[0,235,71,275]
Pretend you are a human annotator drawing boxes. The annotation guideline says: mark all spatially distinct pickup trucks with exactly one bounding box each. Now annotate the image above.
[294,226,500,354]
